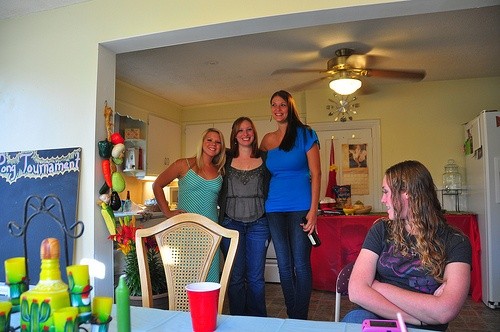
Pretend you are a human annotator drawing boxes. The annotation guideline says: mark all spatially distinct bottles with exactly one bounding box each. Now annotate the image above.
[301,216,321,247]
[19,238,72,332]
[115,276,131,332]
[442,159,464,195]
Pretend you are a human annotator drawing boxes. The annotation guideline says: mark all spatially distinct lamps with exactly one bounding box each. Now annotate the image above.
[330,78,362,96]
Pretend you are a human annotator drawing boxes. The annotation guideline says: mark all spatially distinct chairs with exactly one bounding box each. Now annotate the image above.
[334,261,354,323]
[7,193,84,283]
[135,212,239,315]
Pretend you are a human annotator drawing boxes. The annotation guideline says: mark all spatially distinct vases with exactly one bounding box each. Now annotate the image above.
[129,294,170,311]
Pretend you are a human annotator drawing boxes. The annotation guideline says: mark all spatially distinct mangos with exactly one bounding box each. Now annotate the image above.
[111,171,126,192]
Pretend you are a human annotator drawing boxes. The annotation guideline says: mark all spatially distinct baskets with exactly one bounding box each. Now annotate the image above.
[145,201,169,212]
[354,206,372,214]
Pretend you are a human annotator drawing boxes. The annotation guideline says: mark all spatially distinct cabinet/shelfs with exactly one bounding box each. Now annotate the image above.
[147,116,276,176]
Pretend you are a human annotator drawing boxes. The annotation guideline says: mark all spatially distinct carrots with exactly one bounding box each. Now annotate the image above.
[102,160,110,187]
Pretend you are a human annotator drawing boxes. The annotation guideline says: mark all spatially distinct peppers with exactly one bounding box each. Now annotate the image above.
[98,138,113,157]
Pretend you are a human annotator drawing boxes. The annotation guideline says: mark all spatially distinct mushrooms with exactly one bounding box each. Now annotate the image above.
[111,142,126,159]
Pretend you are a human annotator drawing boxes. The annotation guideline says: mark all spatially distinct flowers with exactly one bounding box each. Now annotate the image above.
[108,216,169,295]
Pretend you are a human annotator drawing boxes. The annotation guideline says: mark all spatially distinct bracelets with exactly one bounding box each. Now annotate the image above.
[420,321,422,325]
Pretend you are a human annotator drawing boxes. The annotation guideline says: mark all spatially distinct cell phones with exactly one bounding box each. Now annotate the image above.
[362,320,400,332]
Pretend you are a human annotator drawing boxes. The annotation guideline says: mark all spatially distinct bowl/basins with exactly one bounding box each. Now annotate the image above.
[355,206,372,214]
[343,208,354,216]
[320,202,336,211]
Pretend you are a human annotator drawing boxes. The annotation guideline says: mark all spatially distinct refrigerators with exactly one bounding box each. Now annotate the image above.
[464,110,500,310]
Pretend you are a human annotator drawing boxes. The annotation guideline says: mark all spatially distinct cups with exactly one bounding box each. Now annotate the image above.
[185,281,222,332]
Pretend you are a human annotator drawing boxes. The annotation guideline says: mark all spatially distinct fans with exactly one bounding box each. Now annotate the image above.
[272,48,427,93]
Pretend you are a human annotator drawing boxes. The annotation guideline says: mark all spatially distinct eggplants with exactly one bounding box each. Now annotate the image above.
[110,192,121,211]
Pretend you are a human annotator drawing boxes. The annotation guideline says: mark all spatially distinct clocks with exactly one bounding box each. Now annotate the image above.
[326,92,360,122]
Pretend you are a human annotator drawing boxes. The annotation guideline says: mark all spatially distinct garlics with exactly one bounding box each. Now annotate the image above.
[99,194,110,204]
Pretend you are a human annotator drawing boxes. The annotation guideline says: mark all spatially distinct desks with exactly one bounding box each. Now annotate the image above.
[0,282,362,332]
[310,211,481,301]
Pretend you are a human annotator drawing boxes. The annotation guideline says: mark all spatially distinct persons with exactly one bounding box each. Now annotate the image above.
[258,90,321,320]
[152,128,227,284]
[218,117,270,317]
[340,160,472,332]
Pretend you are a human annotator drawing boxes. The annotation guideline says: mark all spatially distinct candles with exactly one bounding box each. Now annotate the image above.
[0,300,11,332]
[54,304,79,332]
[92,297,112,323]
[114,274,132,332]
[65,262,92,311]
[5,256,29,305]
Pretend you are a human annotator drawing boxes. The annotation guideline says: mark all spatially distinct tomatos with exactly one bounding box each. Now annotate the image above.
[111,133,124,143]
